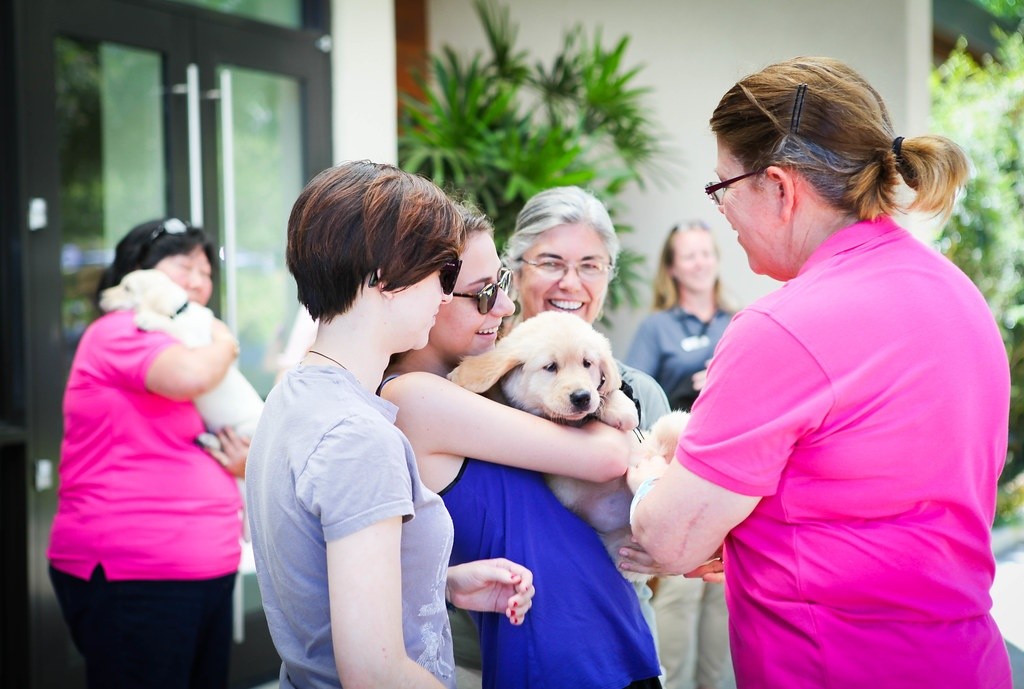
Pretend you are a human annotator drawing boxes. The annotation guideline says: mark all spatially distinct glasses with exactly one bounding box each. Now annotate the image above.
[521,258,611,282]
[438,258,462,295]
[705,163,780,205]
[452,266,511,314]
[136,214,199,264]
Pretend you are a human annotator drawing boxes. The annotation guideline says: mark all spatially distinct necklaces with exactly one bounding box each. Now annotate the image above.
[310,350,349,370]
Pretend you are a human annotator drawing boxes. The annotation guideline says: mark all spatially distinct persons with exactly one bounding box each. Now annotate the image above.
[244,160,536,689]
[46,216,252,689]
[375,187,740,689]
[626,54,1011,689]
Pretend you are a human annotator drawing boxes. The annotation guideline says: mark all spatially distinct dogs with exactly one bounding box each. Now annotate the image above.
[96,267,266,545]
[442,310,693,587]
